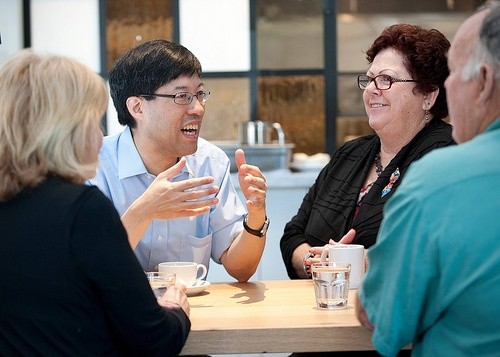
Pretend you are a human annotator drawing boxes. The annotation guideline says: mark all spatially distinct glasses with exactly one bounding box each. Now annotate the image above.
[357,73,415,92]
[141,89,212,106]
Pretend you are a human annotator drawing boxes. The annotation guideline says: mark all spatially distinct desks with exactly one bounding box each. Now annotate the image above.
[177,278,415,356]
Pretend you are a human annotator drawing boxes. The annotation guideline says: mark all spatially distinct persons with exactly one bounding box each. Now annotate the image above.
[242,213,269,238]
[356,0,500,357]
[85,39,266,283]
[279,23,459,357]
[0,48,191,357]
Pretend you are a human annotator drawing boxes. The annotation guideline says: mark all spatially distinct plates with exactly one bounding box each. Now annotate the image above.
[185,280,211,296]
[289,162,326,173]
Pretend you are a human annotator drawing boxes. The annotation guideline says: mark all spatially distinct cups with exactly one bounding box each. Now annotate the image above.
[311,262,351,309]
[237,120,285,145]
[146,271,176,300]
[320,244,365,290]
[158,261,207,286]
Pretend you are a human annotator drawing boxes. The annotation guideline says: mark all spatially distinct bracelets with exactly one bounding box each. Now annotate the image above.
[303,252,310,278]
[306,254,316,278]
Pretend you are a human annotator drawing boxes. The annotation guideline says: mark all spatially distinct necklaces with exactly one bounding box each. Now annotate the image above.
[375,154,382,177]
